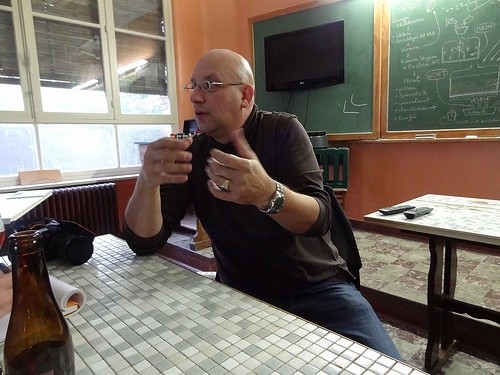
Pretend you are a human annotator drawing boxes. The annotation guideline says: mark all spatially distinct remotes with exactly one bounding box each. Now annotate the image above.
[379,205,416,215]
[403,207,433,219]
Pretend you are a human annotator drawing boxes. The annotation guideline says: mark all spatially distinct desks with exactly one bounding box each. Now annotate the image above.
[0,233,429,375]
[363,193,500,375]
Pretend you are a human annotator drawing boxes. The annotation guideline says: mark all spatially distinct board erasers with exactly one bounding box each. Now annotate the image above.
[414,133,437,140]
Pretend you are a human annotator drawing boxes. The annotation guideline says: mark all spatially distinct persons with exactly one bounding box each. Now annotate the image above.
[124,48,402,361]
[0,271,12,319]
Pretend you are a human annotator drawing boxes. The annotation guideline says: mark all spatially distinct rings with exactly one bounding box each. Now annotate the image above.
[216,180,231,192]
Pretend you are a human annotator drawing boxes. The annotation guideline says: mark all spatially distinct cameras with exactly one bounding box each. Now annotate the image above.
[28,218,94,266]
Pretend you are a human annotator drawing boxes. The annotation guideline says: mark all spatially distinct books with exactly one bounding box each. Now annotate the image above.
[0,275,86,347]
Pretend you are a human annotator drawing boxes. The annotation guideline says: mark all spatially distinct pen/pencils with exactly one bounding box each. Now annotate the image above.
[0,262,12,274]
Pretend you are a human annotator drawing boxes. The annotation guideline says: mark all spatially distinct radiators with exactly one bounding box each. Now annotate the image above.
[0,182,120,256]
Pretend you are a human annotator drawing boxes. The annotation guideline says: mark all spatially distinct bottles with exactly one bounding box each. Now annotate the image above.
[3,229,75,375]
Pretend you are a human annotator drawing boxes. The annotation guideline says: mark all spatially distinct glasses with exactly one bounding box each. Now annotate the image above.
[183,80,243,94]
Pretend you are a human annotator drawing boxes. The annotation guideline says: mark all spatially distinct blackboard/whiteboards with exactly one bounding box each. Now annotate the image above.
[384,0,500,140]
[247,1,380,141]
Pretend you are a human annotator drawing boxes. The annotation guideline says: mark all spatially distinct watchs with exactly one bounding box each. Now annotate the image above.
[259,181,285,214]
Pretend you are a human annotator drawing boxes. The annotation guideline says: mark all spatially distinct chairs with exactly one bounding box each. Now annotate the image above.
[314,147,349,211]
[322,184,362,291]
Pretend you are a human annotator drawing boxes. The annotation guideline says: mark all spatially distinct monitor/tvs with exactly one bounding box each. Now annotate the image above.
[264,20,345,92]
